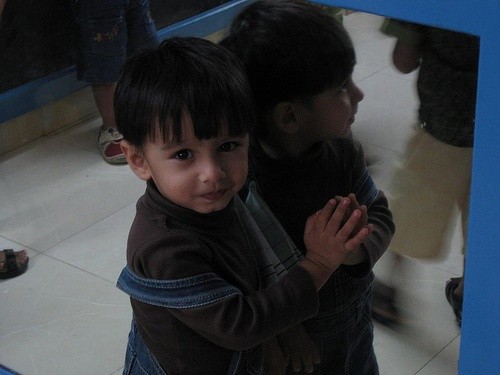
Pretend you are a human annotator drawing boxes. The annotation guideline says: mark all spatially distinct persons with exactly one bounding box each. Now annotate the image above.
[0,247,29,280]
[372,17,480,328]
[72,0,160,163]
[113,36,368,375]
[228,0,396,375]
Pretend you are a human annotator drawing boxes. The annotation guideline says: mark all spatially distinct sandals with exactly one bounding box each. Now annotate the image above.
[0,249,29,281]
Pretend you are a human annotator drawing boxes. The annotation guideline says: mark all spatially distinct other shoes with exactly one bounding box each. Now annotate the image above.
[97,125,128,165]
[371,296,400,327]
[444,276,462,327]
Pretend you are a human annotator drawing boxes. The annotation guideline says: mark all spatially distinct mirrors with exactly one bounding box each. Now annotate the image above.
[0,0,500,375]
